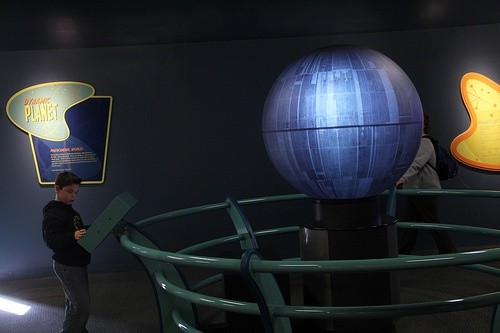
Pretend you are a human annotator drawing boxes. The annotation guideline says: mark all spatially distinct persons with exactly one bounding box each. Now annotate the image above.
[41,171,96,333]
[391,113,461,267]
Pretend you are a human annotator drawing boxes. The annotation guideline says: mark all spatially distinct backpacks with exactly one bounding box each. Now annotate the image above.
[422,136,458,182]
